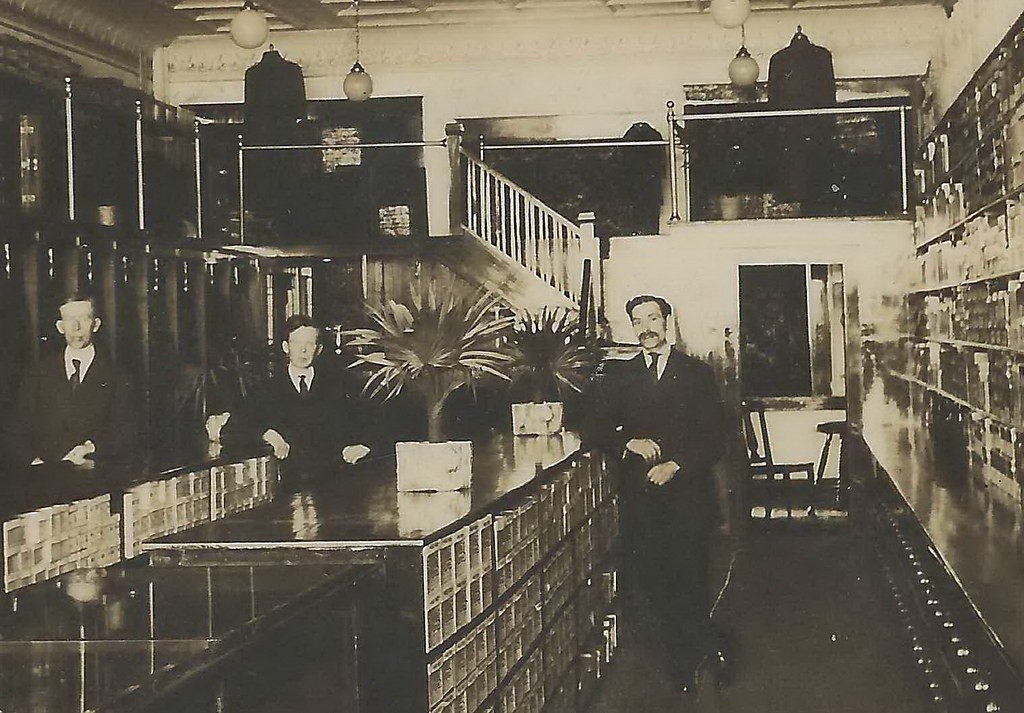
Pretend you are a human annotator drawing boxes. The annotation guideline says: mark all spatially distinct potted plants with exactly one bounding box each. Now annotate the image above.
[324,270,516,492]
[494,304,607,437]
[673,120,781,221]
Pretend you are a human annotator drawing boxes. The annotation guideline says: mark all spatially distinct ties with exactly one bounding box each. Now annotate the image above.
[68,358,81,399]
[298,375,309,399]
[647,352,662,384]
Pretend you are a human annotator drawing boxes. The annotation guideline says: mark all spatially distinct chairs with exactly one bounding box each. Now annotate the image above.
[736,400,813,519]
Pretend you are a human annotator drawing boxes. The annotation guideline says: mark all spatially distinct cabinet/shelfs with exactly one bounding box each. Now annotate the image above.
[855,11,1024,713]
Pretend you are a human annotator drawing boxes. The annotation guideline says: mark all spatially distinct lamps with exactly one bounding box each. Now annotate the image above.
[343,0,373,101]
[709,0,751,28]
[230,0,268,48]
[728,24,759,87]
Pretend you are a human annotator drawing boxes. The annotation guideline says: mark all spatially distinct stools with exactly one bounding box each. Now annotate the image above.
[806,421,848,516]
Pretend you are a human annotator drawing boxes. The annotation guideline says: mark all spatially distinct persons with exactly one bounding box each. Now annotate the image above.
[4,291,146,478]
[576,295,732,701]
[236,315,379,492]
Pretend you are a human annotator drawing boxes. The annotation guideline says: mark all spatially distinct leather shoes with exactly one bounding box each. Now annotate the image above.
[714,639,734,692]
[672,664,694,696]
